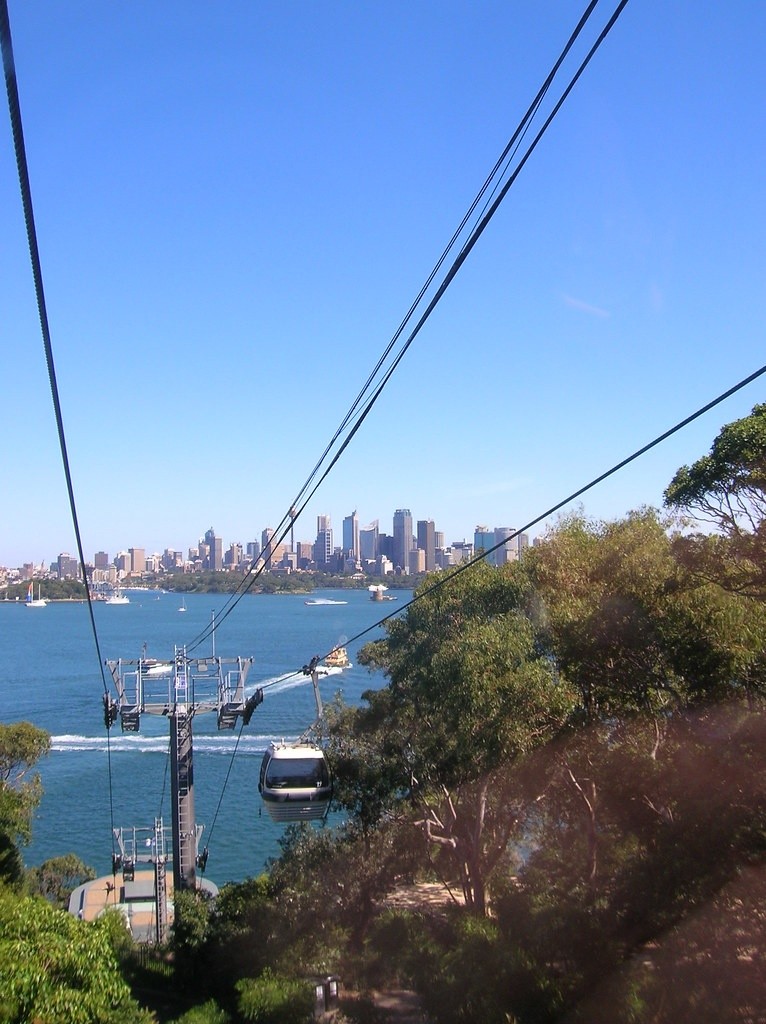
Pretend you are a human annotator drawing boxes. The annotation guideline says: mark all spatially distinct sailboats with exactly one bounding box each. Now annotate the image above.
[24,582,46,607]
[177,597,187,612]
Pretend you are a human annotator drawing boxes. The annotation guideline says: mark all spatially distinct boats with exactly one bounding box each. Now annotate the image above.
[370,594,398,600]
[368,584,389,592]
[106,585,129,604]
[153,594,160,601]
[305,597,348,605]
